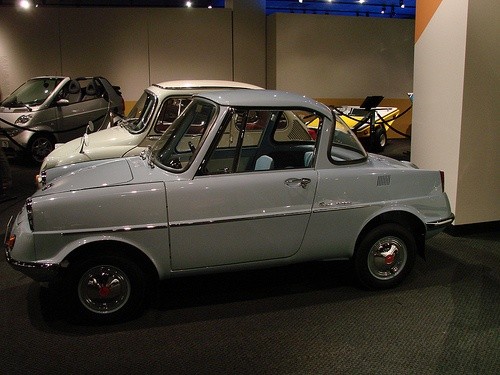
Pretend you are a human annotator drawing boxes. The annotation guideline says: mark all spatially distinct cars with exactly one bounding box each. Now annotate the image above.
[35,79,315,191]
[3,88,456,323]
[0,74,125,165]
